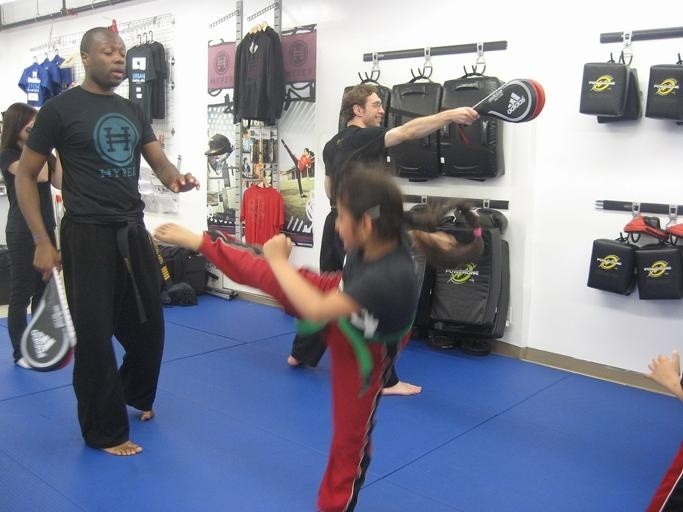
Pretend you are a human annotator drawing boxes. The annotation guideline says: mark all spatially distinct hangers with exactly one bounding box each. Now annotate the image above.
[247,6,268,35]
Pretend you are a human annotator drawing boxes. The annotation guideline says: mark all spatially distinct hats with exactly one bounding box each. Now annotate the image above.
[205,134,229,155]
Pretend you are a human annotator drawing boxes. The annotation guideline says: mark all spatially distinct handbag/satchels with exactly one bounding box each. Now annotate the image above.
[158,245,208,296]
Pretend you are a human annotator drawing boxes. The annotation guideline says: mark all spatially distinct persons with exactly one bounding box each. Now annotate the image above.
[285,83,478,396]
[13,26,202,458]
[152,164,417,512]
[1,99,65,371]
[640,352,680,510]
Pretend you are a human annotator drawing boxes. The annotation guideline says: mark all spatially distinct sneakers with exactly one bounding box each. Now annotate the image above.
[301,195,307,198]
[281,139,285,144]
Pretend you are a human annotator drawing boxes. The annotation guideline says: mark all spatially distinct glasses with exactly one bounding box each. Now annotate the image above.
[368,102,383,108]
